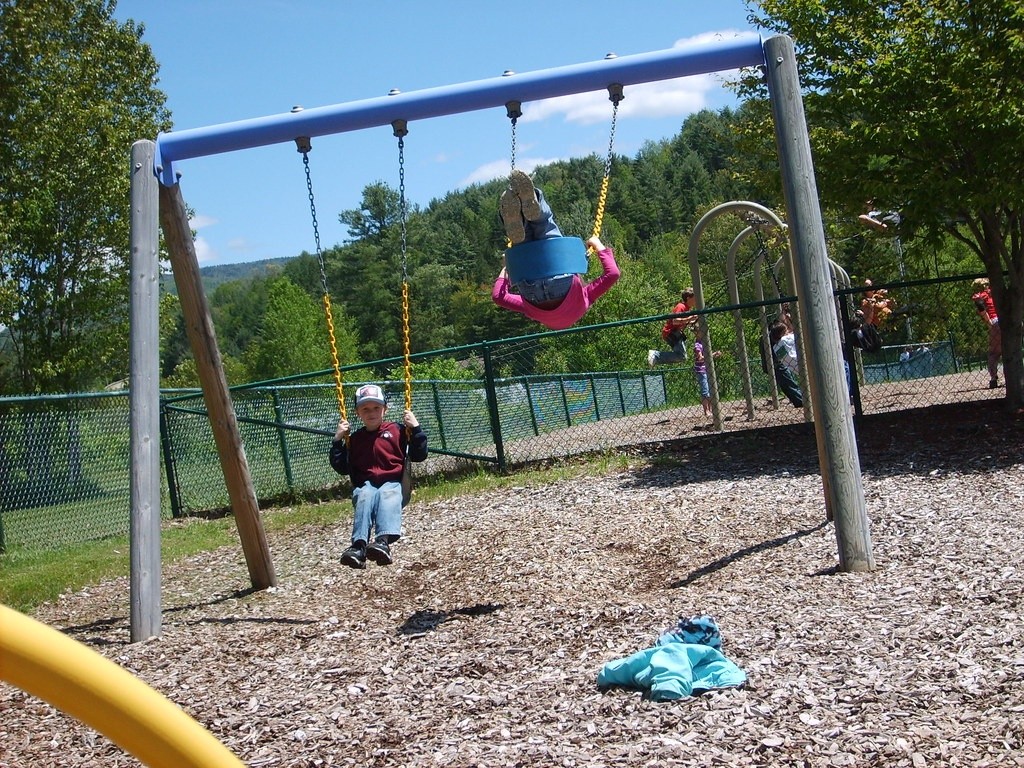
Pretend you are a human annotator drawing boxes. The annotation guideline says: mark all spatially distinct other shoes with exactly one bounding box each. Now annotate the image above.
[989,379,1003,388]
[705,413,714,419]
[498,190,526,244]
[508,171,541,221]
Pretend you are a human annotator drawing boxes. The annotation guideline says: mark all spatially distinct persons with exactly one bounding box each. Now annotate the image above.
[860,202,901,233]
[971,279,1003,387]
[759,279,929,408]
[492,170,621,330]
[648,291,698,369]
[691,323,721,417]
[330,385,428,569]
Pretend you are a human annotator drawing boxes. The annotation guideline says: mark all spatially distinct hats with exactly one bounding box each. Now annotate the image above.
[355,385,387,409]
[971,278,990,293]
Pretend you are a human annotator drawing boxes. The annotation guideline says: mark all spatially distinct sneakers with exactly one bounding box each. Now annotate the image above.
[365,539,392,566]
[341,540,368,570]
[647,349,659,367]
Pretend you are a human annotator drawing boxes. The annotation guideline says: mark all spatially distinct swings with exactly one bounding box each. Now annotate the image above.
[833,275,899,331]
[298,122,415,507]
[491,84,624,326]
[747,211,799,378]
[666,246,763,348]
